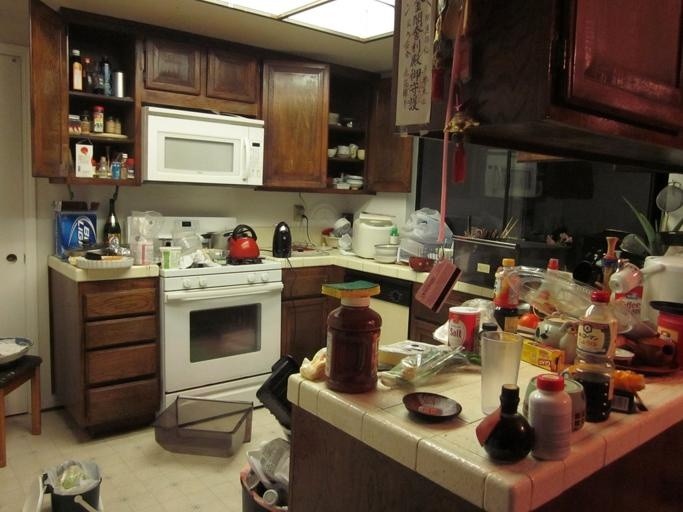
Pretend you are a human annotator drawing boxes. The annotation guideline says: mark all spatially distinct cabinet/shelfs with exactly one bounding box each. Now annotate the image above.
[31,0,141,184]
[79,276,161,428]
[145,34,203,97]
[265,60,371,190]
[207,40,258,105]
[282,267,329,355]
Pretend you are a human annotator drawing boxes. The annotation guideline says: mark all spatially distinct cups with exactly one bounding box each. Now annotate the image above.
[481,332,524,415]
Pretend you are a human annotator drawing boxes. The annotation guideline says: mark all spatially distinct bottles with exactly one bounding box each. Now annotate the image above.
[529,257,559,323]
[83,56,94,94]
[91,159,97,177]
[95,62,105,94]
[447,306,481,355]
[602,236,619,287]
[99,155,108,179]
[324,295,383,395]
[79,114,91,136]
[127,169,136,181]
[101,54,113,97]
[476,321,500,358]
[475,383,534,465]
[528,373,573,461]
[111,68,128,99]
[104,199,121,257]
[69,48,85,93]
[389,226,400,245]
[115,117,122,135]
[67,114,81,137]
[105,115,116,134]
[574,291,617,423]
[111,162,123,180]
[492,257,518,335]
[92,105,105,135]
[125,152,136,169]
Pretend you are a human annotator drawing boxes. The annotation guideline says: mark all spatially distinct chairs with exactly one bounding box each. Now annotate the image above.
[0,336,45,470]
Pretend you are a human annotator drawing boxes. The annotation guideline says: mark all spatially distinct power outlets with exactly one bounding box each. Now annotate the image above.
[293,204,305,221]
